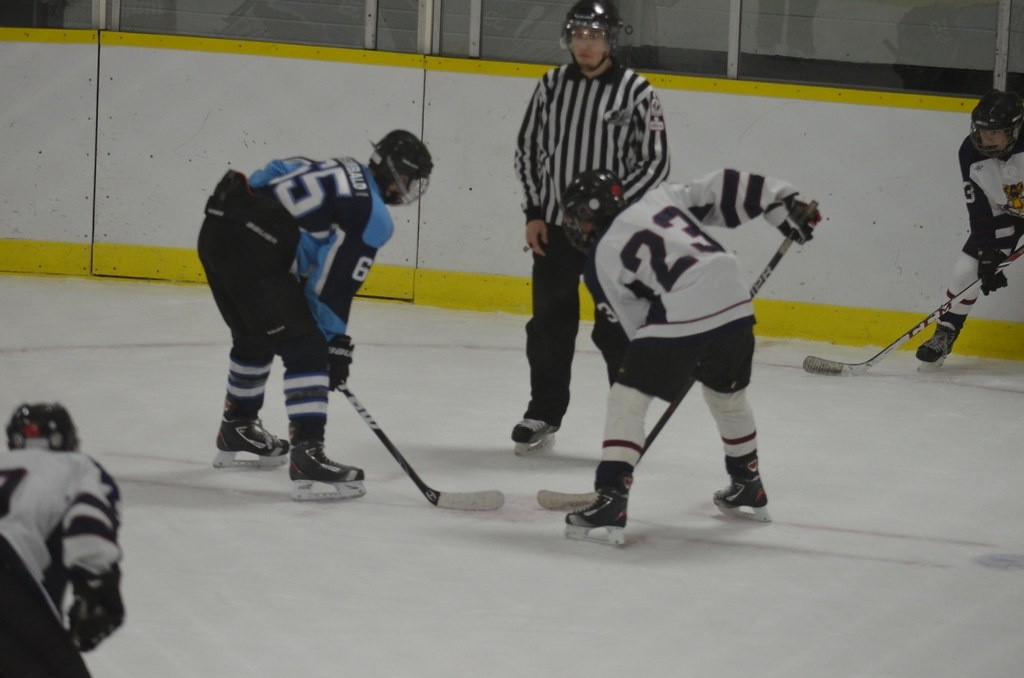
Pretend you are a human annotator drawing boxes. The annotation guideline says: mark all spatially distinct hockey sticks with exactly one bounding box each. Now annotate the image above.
[537,198,820,511]
[339,380,506,511]
[802,244,1024,378]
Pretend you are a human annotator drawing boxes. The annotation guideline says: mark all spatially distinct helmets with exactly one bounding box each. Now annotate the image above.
[967,89,1024,158]
[6,400,76,448]
[558,169,621,252]
[370,130,433,205]
[558,0,621,49]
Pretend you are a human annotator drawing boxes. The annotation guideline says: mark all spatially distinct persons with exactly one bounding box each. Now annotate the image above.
[750,0,818,67]
[916,88,1023,363]
[511,0,672,444]
[0,400,125,678]
[559,163,822,529]
[197,129,434,483]
[892,0,1024,89]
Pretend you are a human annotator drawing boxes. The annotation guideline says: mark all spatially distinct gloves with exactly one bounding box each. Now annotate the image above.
[66,579,125,656]
[976,255,1007,296]
[777,200,821,246]
[325,334,354,388]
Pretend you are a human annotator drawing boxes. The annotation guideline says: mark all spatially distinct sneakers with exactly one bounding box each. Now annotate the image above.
[916,315,962,373]
[213,392,289,468]
[712,456,768,521]
[565,472,632,545]
[510,419,562,454]
[289,419,365,500]
